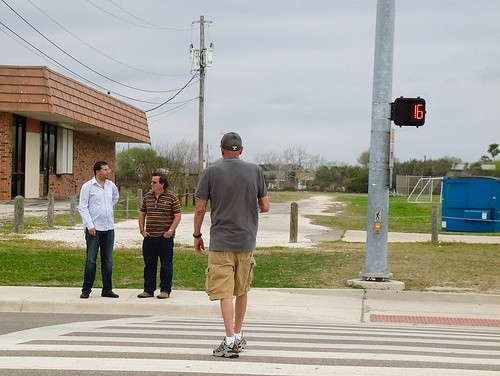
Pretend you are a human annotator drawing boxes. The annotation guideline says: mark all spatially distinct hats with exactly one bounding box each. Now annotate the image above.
[221,132,242,151]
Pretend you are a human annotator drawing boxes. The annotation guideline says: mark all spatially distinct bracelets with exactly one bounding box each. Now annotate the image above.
[140,230,144,233]
[168,229,173,233]
[193,233,202,238]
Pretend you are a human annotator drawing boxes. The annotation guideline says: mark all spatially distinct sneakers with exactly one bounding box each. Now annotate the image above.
[235,337,246,352]
[213,338,239,358]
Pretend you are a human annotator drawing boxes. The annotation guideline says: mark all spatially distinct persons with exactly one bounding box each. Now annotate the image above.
[78,161,120,298]
[194,132,270,358]
[137,172,182,299]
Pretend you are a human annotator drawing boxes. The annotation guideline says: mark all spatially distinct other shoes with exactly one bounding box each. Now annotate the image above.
[101,291,119,298]
[157,291,168,298]
[138,291,154,298]
[80,292,88,298]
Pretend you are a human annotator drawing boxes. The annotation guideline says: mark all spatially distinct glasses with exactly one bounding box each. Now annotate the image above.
[152,182,162,184]
